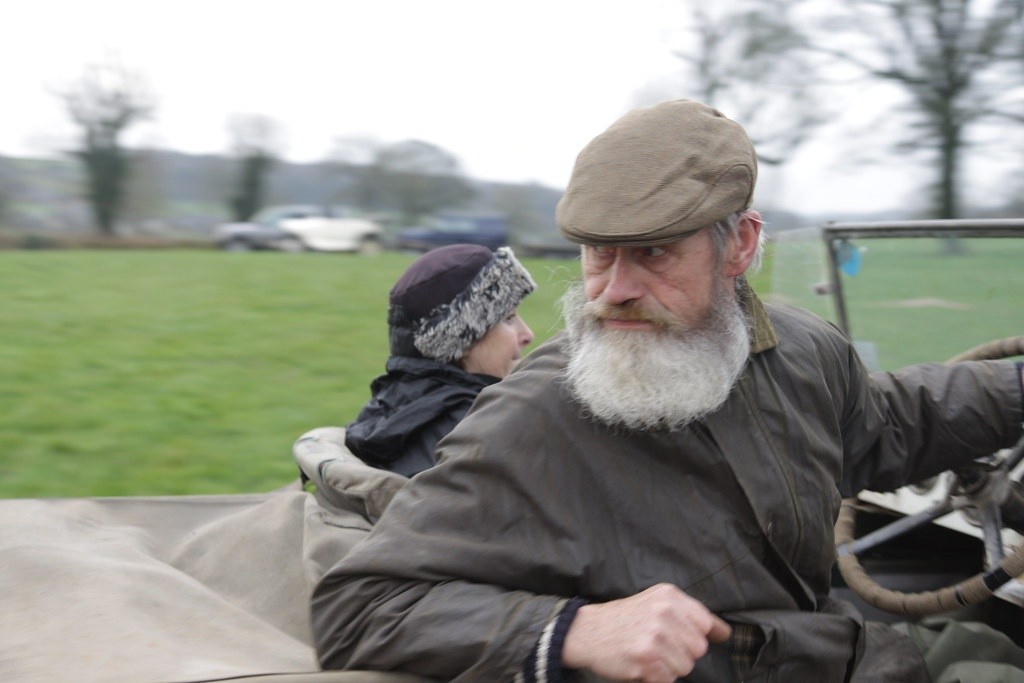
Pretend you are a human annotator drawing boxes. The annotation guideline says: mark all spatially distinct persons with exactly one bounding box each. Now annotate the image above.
[345,243,536,478]
[309,101,1024,683]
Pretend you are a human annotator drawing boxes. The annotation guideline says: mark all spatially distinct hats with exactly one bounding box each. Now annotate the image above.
[555,100,760,247]
[388,245,539,363]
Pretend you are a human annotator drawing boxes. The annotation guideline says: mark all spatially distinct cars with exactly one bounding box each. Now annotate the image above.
[213,202,515,259]
